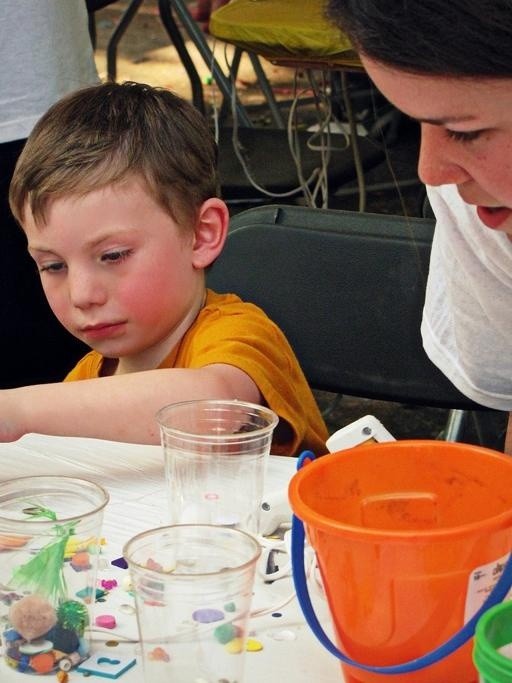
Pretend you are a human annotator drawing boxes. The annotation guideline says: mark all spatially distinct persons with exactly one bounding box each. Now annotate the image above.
[325,2,512,459]
[0,0,102,390]
[0,81,330,458]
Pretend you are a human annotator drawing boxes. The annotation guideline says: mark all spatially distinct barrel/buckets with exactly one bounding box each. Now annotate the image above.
[287,441,512,683]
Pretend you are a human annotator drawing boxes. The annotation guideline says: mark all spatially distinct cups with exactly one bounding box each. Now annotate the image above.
[125,524,262,683]
[155,401,278,540]
[0,473,110,676]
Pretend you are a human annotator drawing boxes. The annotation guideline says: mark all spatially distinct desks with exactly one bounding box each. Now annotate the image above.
[1,431,348,683]
[209,2,370,215]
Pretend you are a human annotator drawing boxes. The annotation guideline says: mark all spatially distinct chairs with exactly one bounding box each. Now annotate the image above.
[88,0,382,209]
[205,203,486,443]
